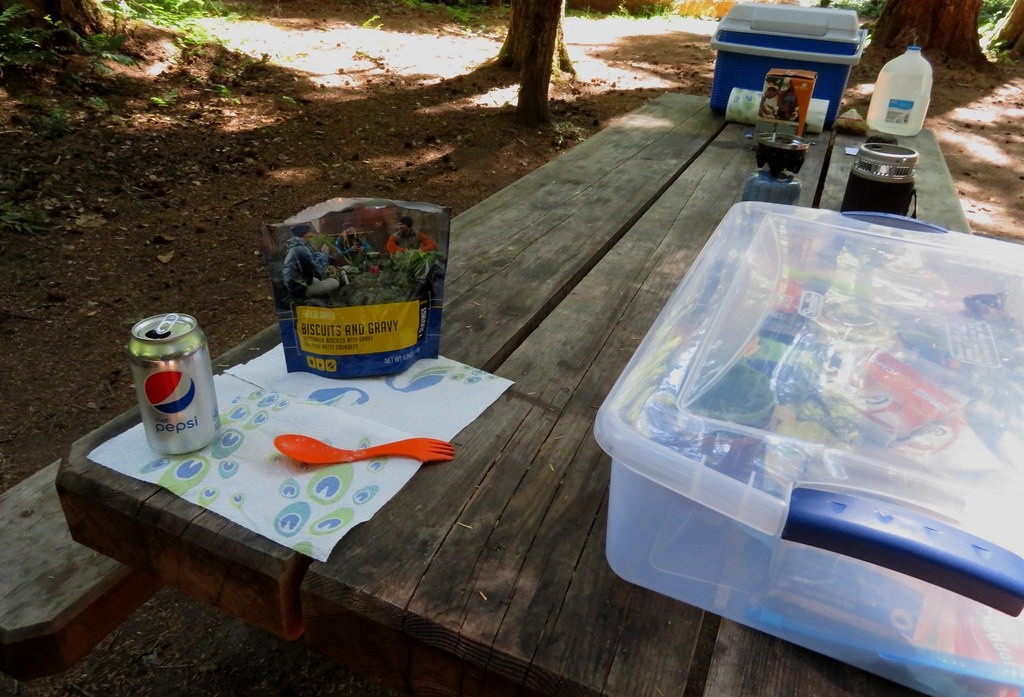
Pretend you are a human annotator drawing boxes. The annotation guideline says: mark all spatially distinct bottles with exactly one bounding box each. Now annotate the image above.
[864,47,934,136]
[840,143,920,216]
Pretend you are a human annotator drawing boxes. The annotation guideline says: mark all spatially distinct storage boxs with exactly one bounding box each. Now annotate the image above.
[581,195,1024,697]
[708,4,868,130]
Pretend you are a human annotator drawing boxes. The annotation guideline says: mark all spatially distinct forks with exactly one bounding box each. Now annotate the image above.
[274,433,456,465]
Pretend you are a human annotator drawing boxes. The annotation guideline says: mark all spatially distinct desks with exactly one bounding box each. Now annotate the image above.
[53,95,970,697]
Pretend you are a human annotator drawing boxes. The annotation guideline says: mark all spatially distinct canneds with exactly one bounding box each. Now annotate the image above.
[127,312,221,455]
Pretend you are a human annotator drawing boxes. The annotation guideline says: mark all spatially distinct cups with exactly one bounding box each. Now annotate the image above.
[725,87,830,134]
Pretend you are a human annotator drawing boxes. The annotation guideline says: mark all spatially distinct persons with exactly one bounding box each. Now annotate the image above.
[336,223,372,271]
[759,87,778,118]
[282,225,339,301]
[387,216,437,261]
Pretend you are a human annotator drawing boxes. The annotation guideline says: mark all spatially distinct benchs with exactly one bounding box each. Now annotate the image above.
[0,460,167,685]
